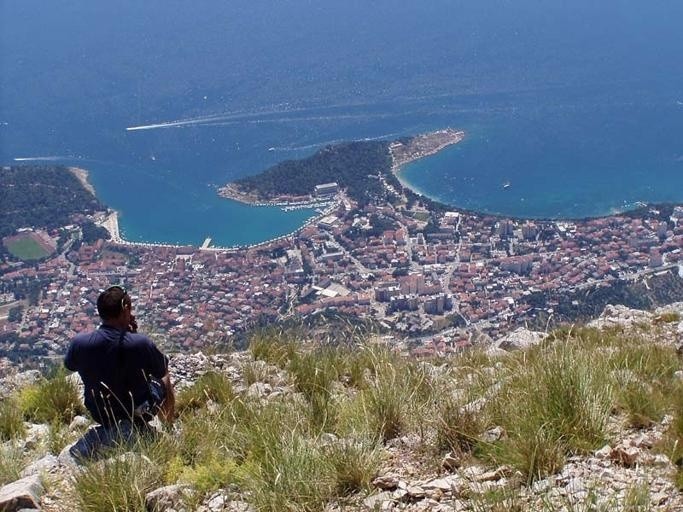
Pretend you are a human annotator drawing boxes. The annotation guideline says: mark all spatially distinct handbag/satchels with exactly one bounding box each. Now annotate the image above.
[84,370,115,423]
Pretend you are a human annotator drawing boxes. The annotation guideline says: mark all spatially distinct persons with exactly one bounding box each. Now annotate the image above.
[63,287,176,433]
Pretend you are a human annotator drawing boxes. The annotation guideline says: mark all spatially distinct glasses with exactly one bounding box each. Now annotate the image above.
[105,284,127,311]
